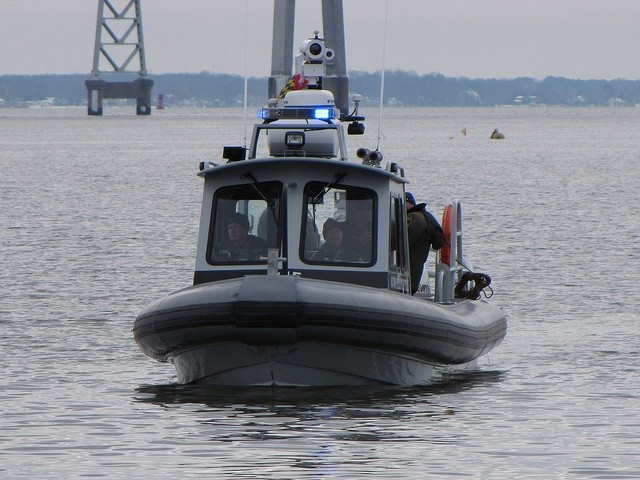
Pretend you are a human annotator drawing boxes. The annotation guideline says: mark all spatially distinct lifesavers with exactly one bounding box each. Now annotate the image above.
[440,205,451,268]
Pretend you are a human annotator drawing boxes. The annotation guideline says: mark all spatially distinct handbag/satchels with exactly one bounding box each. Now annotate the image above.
[422,210,446,250]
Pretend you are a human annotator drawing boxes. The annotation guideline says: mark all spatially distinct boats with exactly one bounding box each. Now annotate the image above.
[132,31,509,391]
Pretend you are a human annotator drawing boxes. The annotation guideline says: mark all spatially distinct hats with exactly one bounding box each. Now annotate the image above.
[323,218,343,234]
[227,212,249,231]
[406,192,416,205]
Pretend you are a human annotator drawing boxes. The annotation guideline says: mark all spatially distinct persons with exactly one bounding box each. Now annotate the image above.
[311,218,363,262]
[213,213,268,259]
[392,193,433,296]
